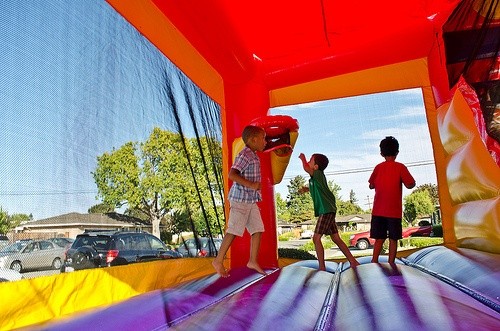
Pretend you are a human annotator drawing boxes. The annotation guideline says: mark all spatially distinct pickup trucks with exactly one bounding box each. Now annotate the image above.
[301,230,314,239]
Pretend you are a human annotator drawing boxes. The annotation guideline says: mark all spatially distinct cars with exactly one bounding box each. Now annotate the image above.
[0,268,25,283]
[176,237,223,258]
[349,224,431,250]
[0,240,69,273]
[48,237,74,248]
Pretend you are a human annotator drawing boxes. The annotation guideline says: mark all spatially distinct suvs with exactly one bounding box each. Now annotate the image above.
[60,228,184,274]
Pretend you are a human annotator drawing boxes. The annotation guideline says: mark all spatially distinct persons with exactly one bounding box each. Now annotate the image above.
[212,125,267,278]
[367,136,416,263]
[299,153,360,271]
